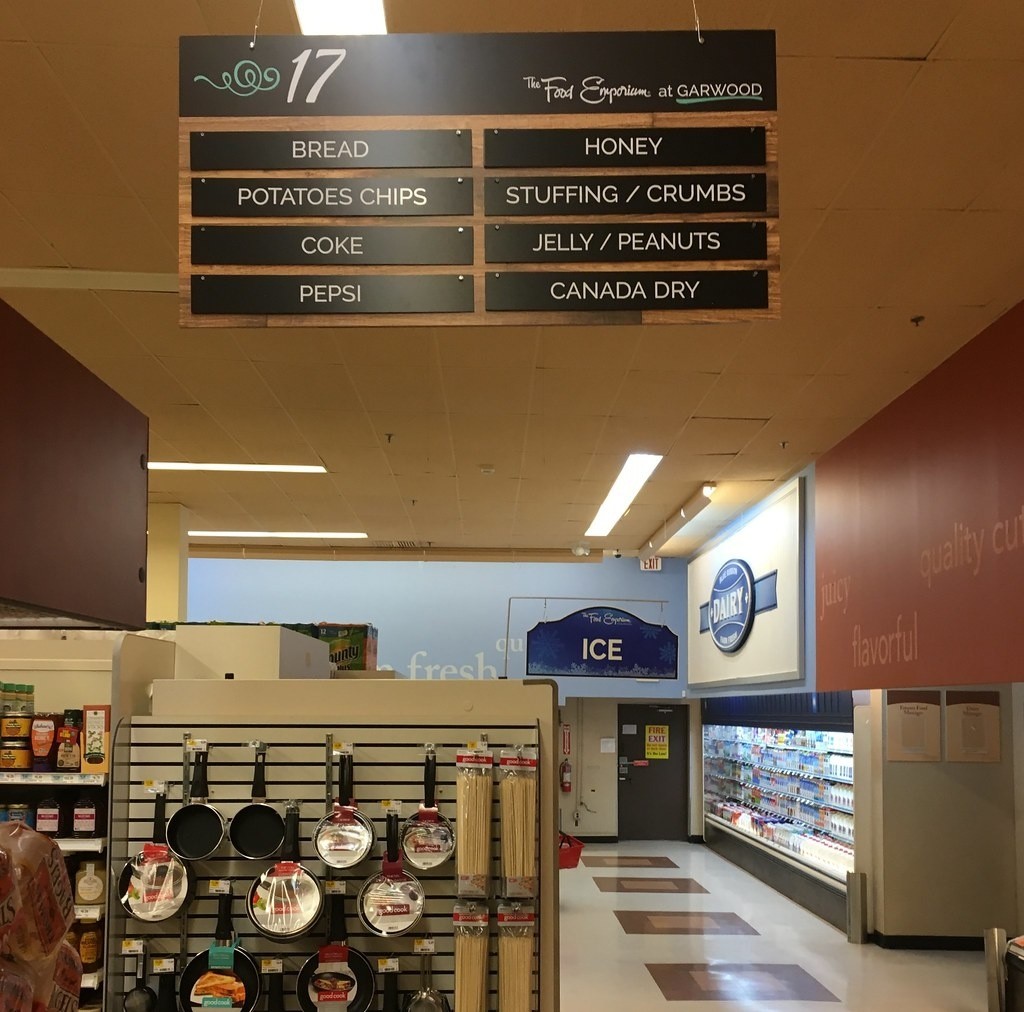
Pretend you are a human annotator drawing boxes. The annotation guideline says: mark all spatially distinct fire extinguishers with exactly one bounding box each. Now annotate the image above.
[558,757,572,792]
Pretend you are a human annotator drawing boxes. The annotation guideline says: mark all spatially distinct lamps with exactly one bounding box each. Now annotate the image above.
[637,481,717,563]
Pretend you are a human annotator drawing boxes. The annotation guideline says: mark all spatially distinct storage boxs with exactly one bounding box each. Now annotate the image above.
[318,622,378,672]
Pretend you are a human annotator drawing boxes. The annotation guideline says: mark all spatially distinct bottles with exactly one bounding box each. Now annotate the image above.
[0,681,34,713]
[715,744,854,882]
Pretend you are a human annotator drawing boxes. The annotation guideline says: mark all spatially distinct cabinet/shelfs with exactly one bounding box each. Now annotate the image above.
[0,631,175,1011]
[701,723,854,934]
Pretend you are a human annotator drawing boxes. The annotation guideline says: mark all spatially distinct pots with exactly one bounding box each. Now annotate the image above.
[245,805,325,943]
[312,751,377,870]
[400,749,456,871]
[357,809,425,938]
[227,741,285,860]
[296,870,375,1012]
[179,882,261,1012]
[117,792,189,923]
[166,751,224,861]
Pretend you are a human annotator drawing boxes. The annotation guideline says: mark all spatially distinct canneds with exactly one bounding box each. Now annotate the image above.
[0,681,107,974]
